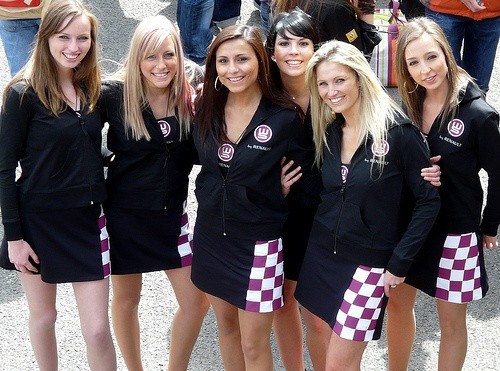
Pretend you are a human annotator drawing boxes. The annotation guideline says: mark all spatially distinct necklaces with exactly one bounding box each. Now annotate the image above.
[288,90,306,101]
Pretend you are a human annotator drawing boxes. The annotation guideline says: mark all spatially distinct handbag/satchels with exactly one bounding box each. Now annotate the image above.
[365,0,408,88]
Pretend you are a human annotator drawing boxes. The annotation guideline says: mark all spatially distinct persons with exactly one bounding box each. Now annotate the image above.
[293,12,441,371]
[281,40,441,371]
[191,7,440,371]
[387,19,500,371]
[0,1,117,371]
[175,0,500,101]
[98,16,210,371]
[0,0,44,79]
